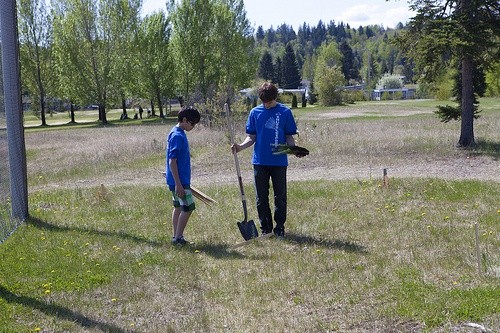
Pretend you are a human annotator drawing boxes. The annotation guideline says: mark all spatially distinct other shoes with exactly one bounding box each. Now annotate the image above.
[274,228,284,237]
[261,231,272,236]
[171,236,195,246]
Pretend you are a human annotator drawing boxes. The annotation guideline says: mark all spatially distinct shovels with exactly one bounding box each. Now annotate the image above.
[225,103,258,242]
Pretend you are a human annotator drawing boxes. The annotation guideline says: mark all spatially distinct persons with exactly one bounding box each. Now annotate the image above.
[231,83,306,243]
[166,107,201,246]
[139,106,144,119]
